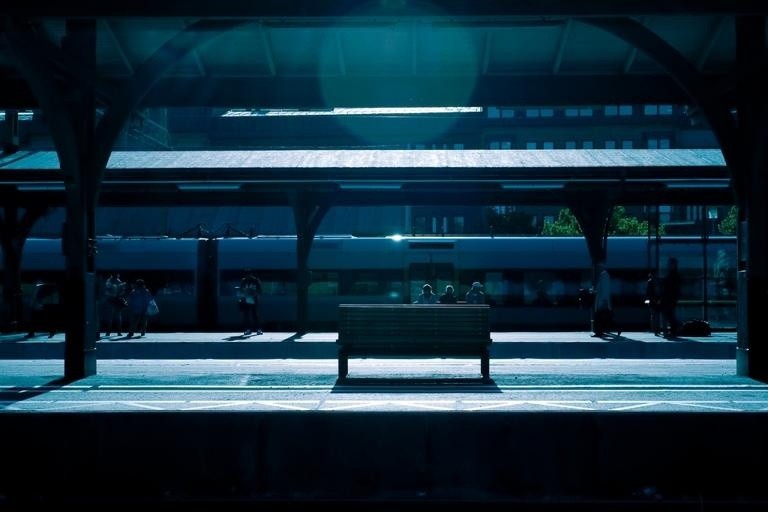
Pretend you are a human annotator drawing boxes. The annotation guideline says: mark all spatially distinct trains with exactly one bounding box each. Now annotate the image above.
[18,235,743,334]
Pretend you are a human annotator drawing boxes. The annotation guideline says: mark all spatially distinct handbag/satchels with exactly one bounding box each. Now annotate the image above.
[145,300,160,316]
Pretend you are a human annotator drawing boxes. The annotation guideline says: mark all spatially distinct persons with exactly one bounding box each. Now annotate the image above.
[23,275,55,339]
[417,283,436,304]
[104,271,128,336]
[658,256,681,338]
[464,281,485,304]
[439,284,457,304]
[588,262,623,338]
[237,270,264,335]
[126,278,152,337]
[645,271,663,337]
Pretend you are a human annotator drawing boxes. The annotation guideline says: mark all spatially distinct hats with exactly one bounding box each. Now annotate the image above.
[472,282,483,287]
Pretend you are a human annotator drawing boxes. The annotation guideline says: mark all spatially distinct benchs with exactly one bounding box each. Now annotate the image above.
[334,304,495,384]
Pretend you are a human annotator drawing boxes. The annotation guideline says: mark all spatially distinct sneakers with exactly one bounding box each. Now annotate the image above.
[618,331,622,336]
[106,333,144,340]
[24,334,34,338]
[257,330,263,335]
[48,335,53,338]
[245,330,250,335]
[591,333,601,337]
